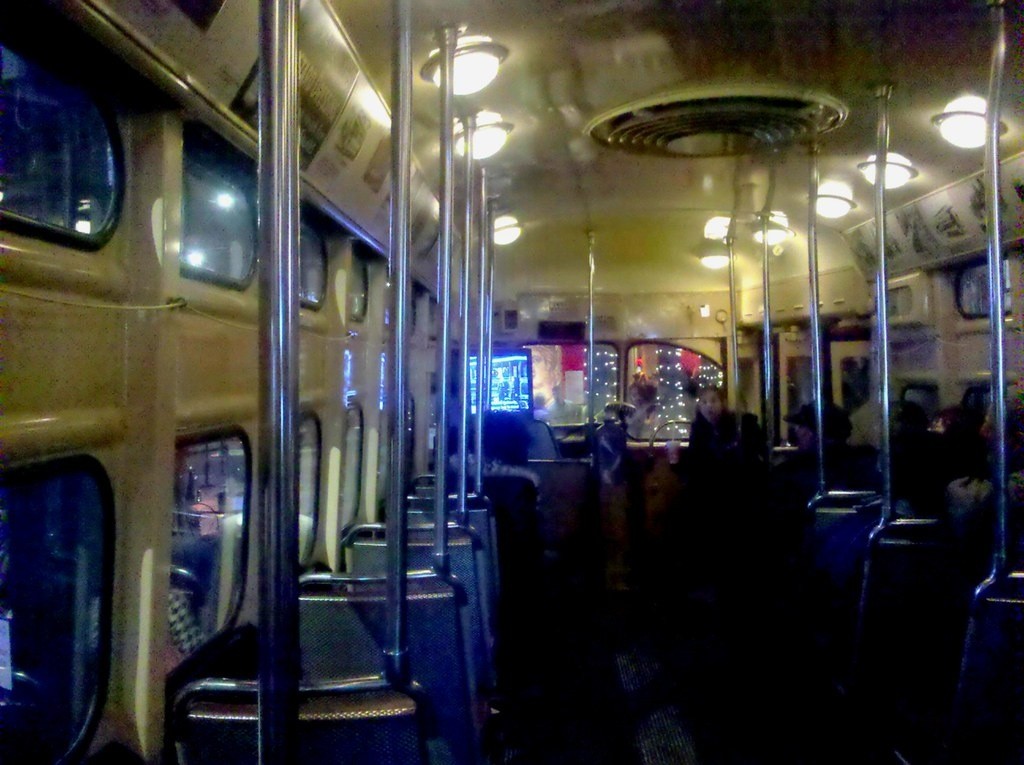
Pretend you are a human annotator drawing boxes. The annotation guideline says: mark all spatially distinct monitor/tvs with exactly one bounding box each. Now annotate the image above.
[448,348,534,426]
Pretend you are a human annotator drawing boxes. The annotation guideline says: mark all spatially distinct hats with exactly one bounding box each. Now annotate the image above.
[783,400,853,440]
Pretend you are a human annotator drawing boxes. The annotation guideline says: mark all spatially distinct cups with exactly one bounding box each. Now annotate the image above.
[667,440,680,464]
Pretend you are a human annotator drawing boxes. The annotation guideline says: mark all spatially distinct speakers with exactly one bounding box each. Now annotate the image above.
[500,307,520,335]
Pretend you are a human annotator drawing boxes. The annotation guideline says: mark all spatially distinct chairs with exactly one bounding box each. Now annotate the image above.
[743,440,1024,765]
[157,410,562,765]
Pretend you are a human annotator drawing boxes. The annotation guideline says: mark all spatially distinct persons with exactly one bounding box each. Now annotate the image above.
[664,385,769,477]
[946,376,1023,571]
[926,403,975,436]
[753,403,882,552]
[448,410,580,549]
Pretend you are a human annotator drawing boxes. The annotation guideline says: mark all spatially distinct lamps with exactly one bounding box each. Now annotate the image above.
[418,33,510,98]
[854,150,921,191]
[749,211,796,247]
[807,181,858,220]
[687,242,739,270]
[929,93,1009,150]
[492,213,524,247]
[453,108,515,161]
